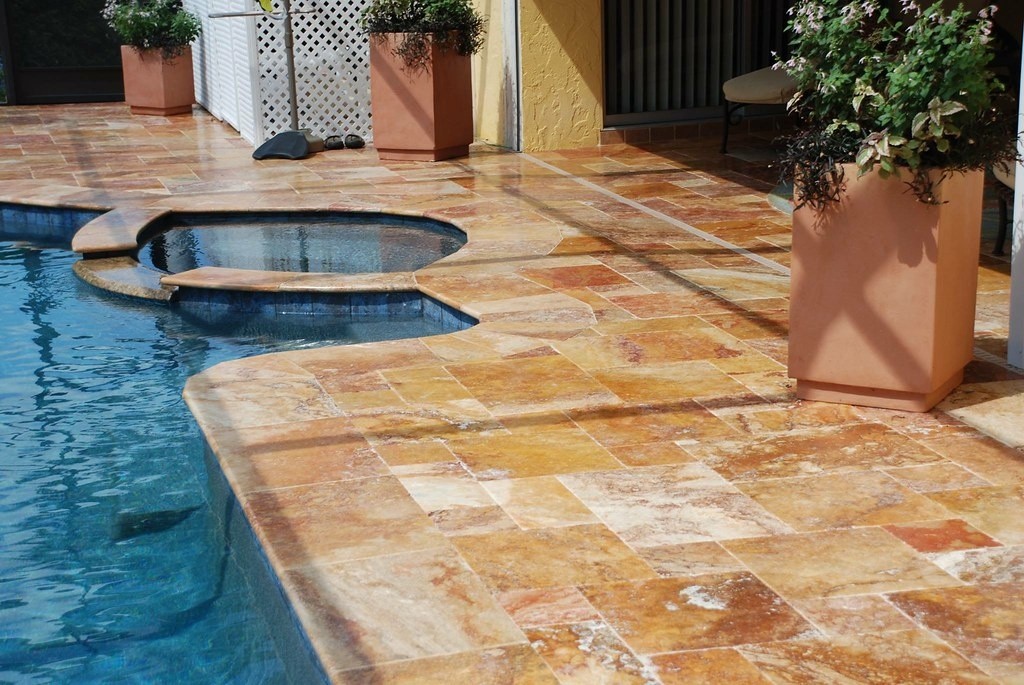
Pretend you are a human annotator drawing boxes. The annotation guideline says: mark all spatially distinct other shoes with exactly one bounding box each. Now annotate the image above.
[345,134,365,149]
[325,134,344,150]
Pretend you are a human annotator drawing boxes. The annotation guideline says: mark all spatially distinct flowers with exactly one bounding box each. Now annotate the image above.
[98,0,202,68]
[769,1,1024,211]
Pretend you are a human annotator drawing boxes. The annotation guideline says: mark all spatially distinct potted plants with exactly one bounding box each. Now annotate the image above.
[359,1,490,163]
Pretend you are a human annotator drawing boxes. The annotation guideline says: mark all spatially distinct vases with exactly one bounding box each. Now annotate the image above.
[787,163,986,414]
[120,44,195,116]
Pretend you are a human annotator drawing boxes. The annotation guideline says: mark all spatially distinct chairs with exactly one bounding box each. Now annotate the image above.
[723,64,808,152]
[993,141,1017,254]
